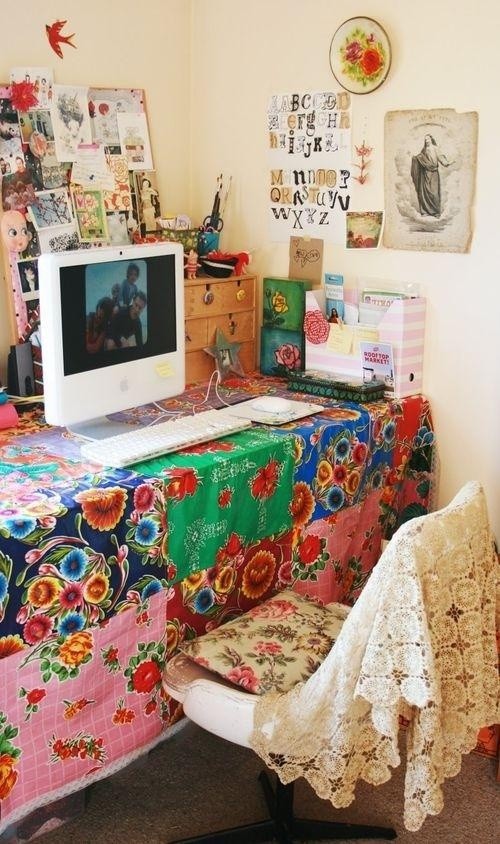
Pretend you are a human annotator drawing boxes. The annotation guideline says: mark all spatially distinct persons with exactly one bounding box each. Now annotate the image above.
[86,296,112,351]
[183,249,202,280]
[103,292,146,351]
[328,308,339,323]
[221,350,232,366]
[405,133,456,219]
[111,263,139,308]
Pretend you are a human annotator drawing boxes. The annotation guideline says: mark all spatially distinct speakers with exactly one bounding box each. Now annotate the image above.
[5,341,36,412]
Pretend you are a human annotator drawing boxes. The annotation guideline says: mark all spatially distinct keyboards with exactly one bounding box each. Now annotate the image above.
[79,407,252,469]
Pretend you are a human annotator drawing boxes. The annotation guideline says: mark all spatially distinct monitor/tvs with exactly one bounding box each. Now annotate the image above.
[36,239,186,445]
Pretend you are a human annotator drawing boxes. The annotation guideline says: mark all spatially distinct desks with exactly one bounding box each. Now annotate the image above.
[0,374,440,833]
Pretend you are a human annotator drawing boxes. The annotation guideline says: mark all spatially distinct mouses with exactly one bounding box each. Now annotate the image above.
[253,394,294,415]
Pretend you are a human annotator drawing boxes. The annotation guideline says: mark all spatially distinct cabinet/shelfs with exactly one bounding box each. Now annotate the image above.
[184,273,259,385]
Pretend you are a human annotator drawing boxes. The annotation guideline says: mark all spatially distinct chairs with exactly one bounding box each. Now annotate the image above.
[157,481,485,844]
